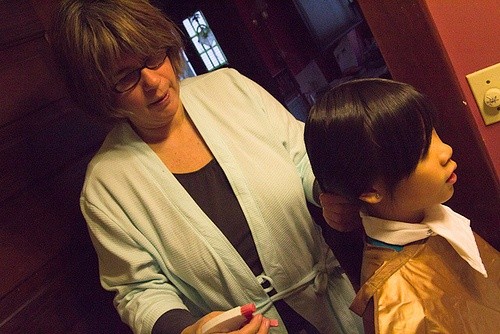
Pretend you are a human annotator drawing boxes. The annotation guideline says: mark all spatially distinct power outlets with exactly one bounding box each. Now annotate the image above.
[465,62,500,126]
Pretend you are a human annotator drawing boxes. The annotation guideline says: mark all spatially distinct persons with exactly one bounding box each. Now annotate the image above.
[54,0,362,334]
[304,76,499,334]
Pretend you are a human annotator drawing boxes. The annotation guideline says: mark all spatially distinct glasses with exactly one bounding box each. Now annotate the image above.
[111,48,167,93]
